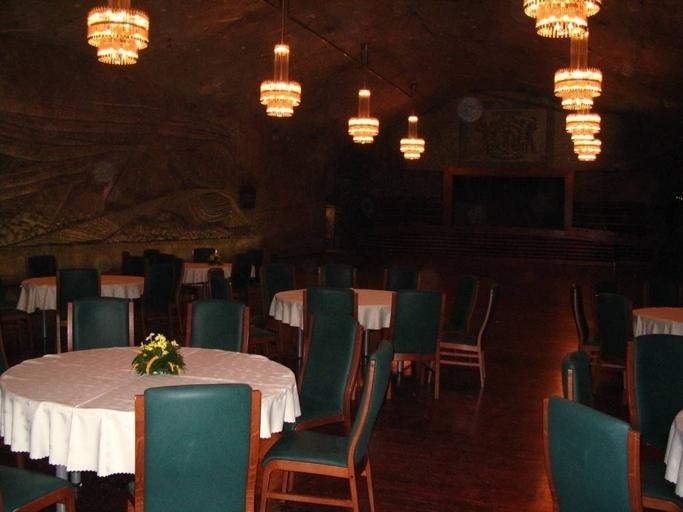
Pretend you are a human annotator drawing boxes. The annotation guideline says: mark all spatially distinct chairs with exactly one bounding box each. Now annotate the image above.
[2,247,502,337]
[428,280,496,389]
[67,296,135,351]
[127,384,261,512]
[542,249,683,512]
[260,339,394,512]
[280,309,365,505]
[393,288,449,402]
[2,464,77,512]
[185,299,251,353]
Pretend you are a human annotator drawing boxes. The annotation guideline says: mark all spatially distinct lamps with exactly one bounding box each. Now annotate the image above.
[400,82,426,160]
[86,1,151,67]
[347,43,380,145]
[524,0,604,164]
[258,0,302,120]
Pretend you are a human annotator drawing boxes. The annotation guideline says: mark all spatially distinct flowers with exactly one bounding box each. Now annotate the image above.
[131,332,187,377]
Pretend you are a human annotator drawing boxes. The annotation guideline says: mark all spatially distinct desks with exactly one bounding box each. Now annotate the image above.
[1,345,303,512]
[269,290,395,358]
